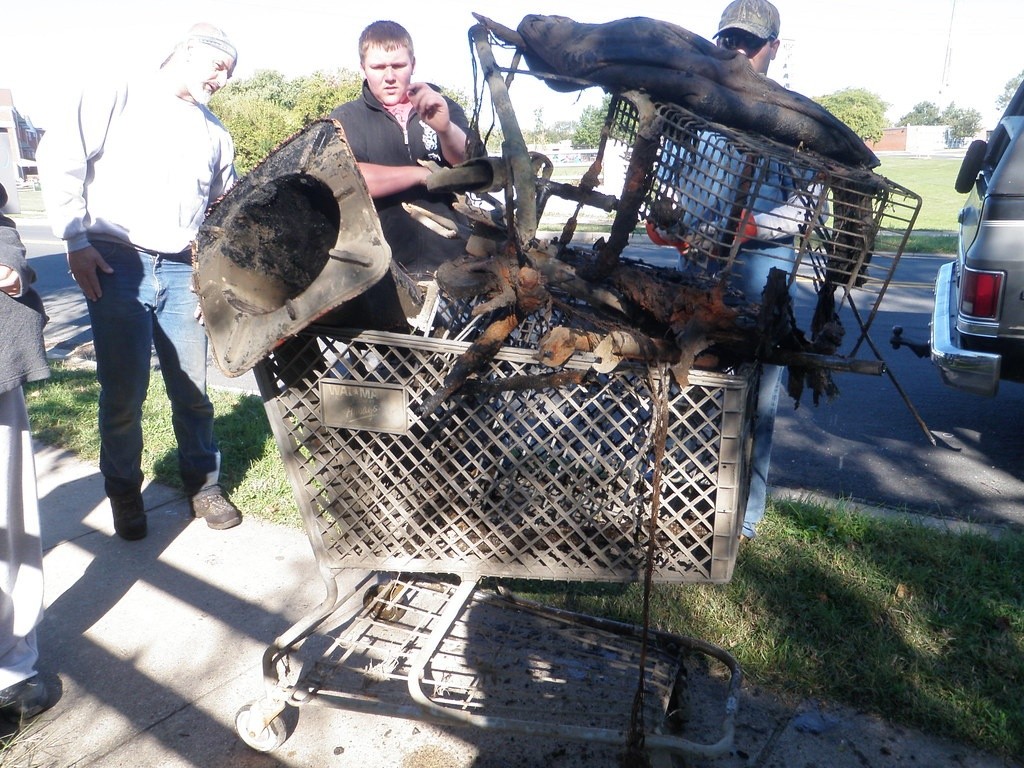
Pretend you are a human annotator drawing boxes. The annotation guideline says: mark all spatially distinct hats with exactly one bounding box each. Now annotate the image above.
[711,0,780,41]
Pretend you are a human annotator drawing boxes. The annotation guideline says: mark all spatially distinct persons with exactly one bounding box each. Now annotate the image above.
[0,178,50,723]
[35,21,243,540]
[644,1,831,555]
[324,20,490,285]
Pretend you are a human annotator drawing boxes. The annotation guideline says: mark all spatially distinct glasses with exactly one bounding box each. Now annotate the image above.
[717,33,775,50]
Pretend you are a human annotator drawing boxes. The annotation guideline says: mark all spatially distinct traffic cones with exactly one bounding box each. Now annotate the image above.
[192,119,448,379]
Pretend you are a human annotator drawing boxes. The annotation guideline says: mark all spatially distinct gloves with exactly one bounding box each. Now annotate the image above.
[678,209,757,263]
[647,219,686,247]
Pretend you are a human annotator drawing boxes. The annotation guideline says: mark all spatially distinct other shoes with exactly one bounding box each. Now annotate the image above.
[0,673,62,726]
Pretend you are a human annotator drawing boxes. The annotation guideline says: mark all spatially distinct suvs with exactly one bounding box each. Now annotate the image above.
[889,79,1024,398]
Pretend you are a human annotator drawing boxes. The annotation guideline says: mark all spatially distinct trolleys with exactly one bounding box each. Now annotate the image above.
[235,289,763,768]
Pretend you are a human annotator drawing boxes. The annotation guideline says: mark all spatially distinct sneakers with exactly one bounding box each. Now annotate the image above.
[110,493,147,540]
[190,493,242,530]
